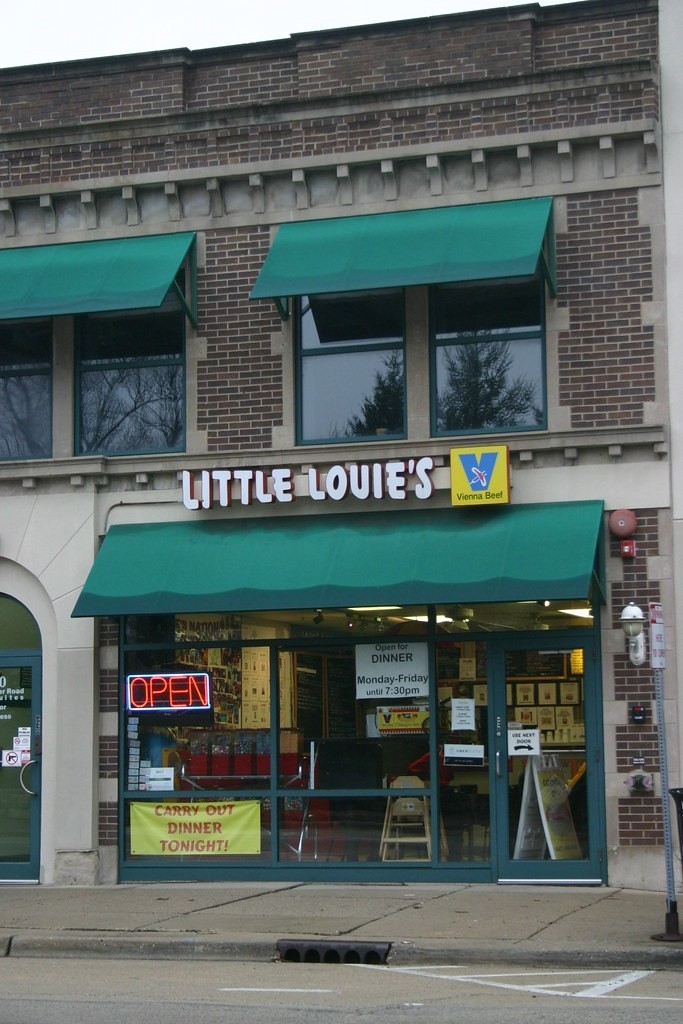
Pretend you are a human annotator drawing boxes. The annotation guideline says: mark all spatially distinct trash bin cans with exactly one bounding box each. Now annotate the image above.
[667,787,682,864]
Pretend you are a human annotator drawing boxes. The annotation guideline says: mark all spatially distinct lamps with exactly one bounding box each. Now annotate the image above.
[619,600,645,666]
[313,612,325,625]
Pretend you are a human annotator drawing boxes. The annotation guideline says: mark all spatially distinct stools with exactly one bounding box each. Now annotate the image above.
[381,776,449,863]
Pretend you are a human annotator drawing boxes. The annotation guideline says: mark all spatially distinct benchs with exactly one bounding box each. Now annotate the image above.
[180,757,319,861]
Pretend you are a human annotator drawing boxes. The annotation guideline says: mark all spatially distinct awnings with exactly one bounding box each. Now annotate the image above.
[70,499,605,616]
[0,231,197,327]
[248,195,558,300]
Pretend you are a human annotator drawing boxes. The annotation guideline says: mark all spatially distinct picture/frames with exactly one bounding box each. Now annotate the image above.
[292,650,325,740]
[324,655,359,739]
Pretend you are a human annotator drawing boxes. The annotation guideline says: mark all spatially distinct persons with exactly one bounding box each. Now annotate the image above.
[422,718,431,734]
